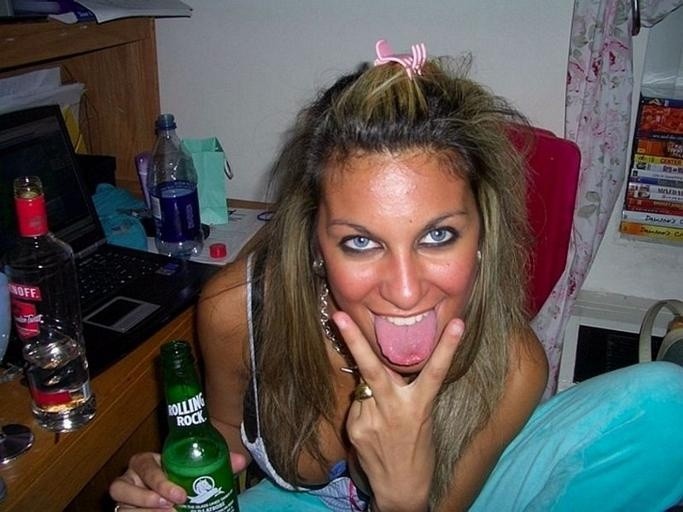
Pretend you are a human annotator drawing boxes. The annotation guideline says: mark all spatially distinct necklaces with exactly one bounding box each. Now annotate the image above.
[312,277,368,377]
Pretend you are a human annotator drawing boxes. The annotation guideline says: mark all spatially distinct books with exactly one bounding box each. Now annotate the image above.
[617,87,682,249]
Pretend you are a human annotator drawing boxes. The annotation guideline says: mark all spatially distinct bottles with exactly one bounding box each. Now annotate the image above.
[7,173,96,433]
[153,335,237,512]
[146,111,201,259]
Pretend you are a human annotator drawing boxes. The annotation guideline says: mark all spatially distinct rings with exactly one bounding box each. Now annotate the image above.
[113,503,123,512]
[351,382,373,402]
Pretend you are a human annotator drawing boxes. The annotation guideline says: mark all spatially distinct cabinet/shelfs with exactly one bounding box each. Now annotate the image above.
[557,289,679,401]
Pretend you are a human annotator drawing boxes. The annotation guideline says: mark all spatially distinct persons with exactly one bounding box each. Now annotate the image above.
[106,39,551,512]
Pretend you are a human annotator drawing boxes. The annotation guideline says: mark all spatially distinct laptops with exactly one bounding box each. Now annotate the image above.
[0,104,201,371]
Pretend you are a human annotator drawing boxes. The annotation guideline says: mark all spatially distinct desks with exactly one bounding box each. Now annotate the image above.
[0,197,285,512]
